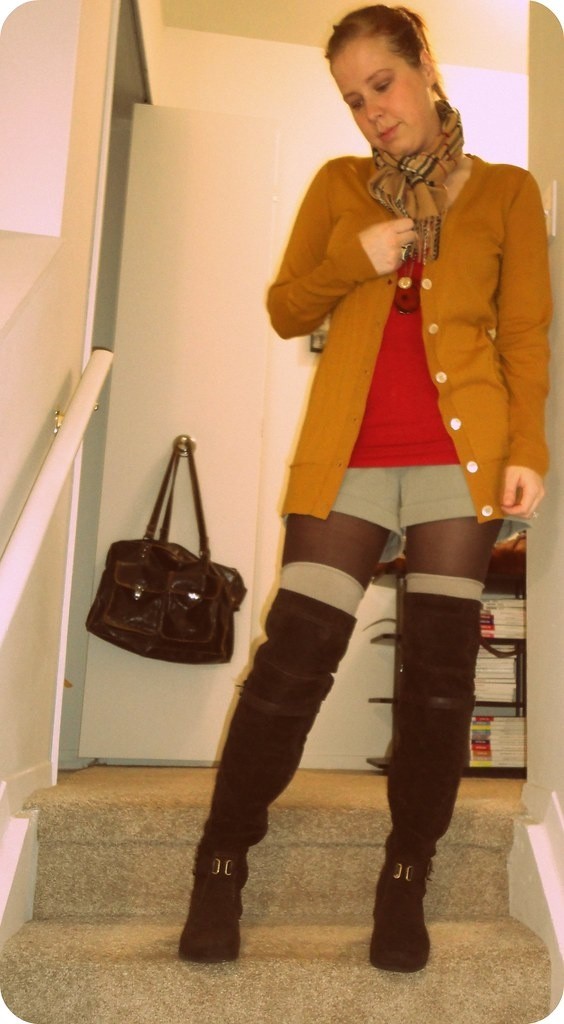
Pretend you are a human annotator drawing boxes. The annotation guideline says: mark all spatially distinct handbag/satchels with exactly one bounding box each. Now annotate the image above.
[84,434,247,664]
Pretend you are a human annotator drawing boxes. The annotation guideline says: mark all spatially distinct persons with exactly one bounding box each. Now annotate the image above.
[178,5,550,973]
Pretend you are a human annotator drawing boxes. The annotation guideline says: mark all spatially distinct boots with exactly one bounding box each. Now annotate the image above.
[369,593,484,973]
[177,588,357,964]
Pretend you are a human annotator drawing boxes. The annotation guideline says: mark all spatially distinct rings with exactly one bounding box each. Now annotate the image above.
[533,512,537,518]
[401,244,412,261]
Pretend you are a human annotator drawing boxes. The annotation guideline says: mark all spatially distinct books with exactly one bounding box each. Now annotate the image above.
[481,598,526,638]
[469,716,528,768]
[474,643,516,702]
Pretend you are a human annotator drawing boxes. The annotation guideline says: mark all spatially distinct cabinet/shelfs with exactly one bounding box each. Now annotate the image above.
[361,549,528,780]
